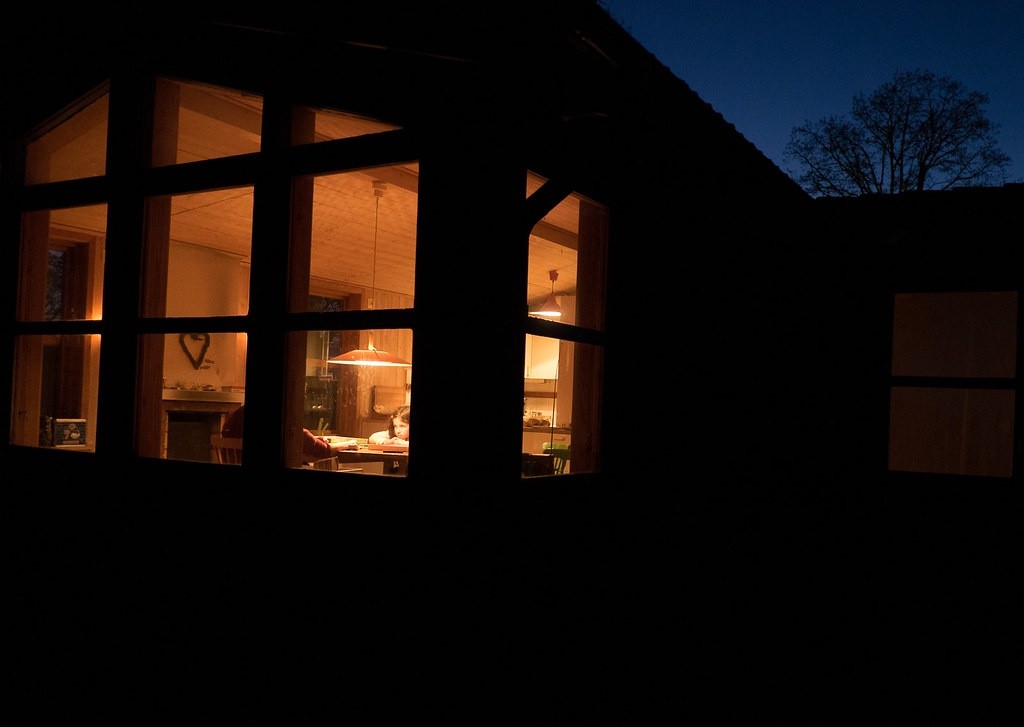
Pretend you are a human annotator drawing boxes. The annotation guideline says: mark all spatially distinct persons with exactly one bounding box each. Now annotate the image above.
[369,406,410,446]
[222,382,360,466]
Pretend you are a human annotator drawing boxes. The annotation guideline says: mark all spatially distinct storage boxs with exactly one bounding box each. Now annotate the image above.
[52,419,86,446]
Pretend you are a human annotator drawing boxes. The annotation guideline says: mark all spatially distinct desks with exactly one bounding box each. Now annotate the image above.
[334,442,409,477]
[522,452,554,477]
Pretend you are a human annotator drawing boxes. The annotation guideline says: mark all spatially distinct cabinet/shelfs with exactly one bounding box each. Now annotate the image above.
[524,316,558,380]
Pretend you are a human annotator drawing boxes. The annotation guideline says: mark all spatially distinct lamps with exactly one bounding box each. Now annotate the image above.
[538,270,562,317]
[325,182,408,368]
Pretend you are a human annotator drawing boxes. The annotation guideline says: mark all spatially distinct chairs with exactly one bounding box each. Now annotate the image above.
[210,434,242,465]
[542,448,571,475]
